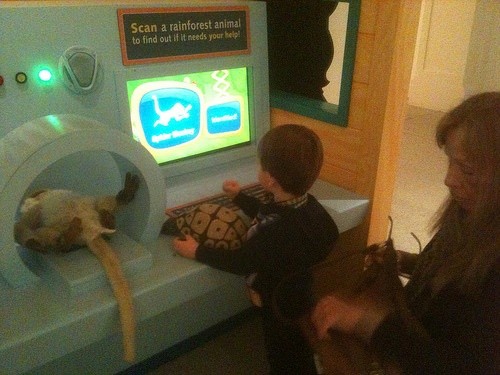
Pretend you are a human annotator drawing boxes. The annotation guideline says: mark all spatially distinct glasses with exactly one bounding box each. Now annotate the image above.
[373,216,423,282]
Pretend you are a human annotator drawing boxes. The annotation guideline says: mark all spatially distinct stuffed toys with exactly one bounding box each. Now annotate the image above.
[175,203,281,254]
[17,172,146,361]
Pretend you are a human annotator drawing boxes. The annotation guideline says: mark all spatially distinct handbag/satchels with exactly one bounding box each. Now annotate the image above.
[271,250,432,374]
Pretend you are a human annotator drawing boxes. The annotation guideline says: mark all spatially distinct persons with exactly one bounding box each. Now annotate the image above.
[308,87,499,374]
[174,124,341,374]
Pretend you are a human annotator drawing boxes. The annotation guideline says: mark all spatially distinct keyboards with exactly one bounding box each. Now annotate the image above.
[164,181,273,221]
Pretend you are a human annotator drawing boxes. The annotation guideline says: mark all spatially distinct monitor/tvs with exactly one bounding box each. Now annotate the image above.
[112,54,262,180]
